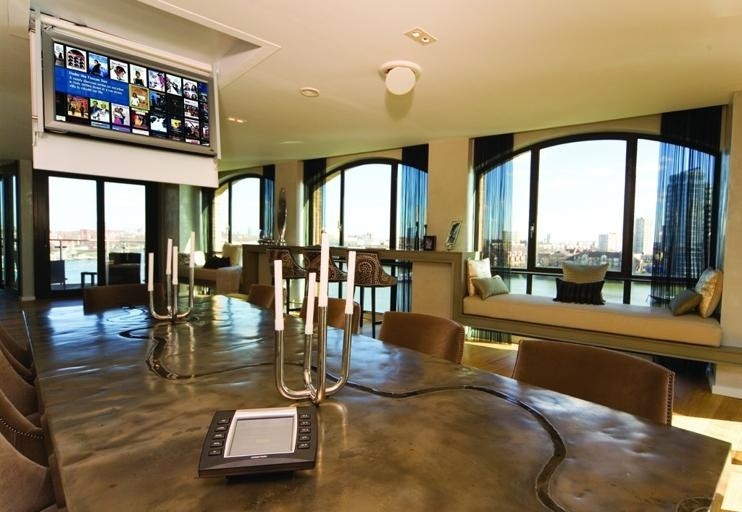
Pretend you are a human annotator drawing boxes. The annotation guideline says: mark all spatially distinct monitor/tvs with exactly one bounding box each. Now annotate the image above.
[41,27,218,158]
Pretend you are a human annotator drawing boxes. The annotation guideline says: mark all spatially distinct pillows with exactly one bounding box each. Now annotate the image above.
[222,242,242,265]
[466,257,723,320]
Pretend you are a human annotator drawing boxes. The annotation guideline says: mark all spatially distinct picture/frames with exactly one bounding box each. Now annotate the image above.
[423,235,436,251]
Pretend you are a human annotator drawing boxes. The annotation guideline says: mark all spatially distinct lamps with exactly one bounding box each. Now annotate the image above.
[379,62,422,96]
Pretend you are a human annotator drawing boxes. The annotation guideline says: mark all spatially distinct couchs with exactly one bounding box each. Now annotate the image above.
[178,253,243,294]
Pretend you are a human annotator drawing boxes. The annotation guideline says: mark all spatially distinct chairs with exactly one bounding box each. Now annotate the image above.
[0,322,68,512]
[83,282,162,314]
[269,248,306,315]
[50,252,140,287]
[299,296,361,334]
[247,285,285,312]
[510,340,675,426]
[303,250,347,299]
[377,312,465,365]
[346,252,397,339]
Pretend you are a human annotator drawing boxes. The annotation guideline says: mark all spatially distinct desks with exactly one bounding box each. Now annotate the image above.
[242,244,482,342]
[22,294,732,512]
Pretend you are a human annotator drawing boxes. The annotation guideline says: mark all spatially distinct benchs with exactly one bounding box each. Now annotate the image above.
[454,251,721,394]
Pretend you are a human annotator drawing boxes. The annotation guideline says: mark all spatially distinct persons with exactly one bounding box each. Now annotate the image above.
[54,49,210,147]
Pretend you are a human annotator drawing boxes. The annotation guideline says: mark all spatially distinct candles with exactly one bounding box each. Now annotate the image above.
[147,238,195,292]
[274,233,356,335]
